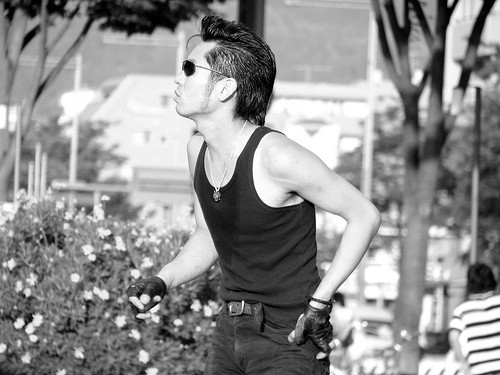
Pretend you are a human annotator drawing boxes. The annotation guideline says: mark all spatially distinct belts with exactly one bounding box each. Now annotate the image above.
[224,299,254,317]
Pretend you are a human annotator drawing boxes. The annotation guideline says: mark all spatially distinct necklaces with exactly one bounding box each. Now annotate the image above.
[206,120,248,202]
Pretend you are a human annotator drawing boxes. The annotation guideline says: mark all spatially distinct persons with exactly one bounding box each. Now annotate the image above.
[125,16,381,375]
[446,262,500,375]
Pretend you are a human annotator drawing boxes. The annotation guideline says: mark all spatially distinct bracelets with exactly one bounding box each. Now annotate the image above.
[307,296,333,307]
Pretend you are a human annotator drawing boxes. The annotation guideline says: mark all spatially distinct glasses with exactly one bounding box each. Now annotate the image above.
[181,60,229,76]
